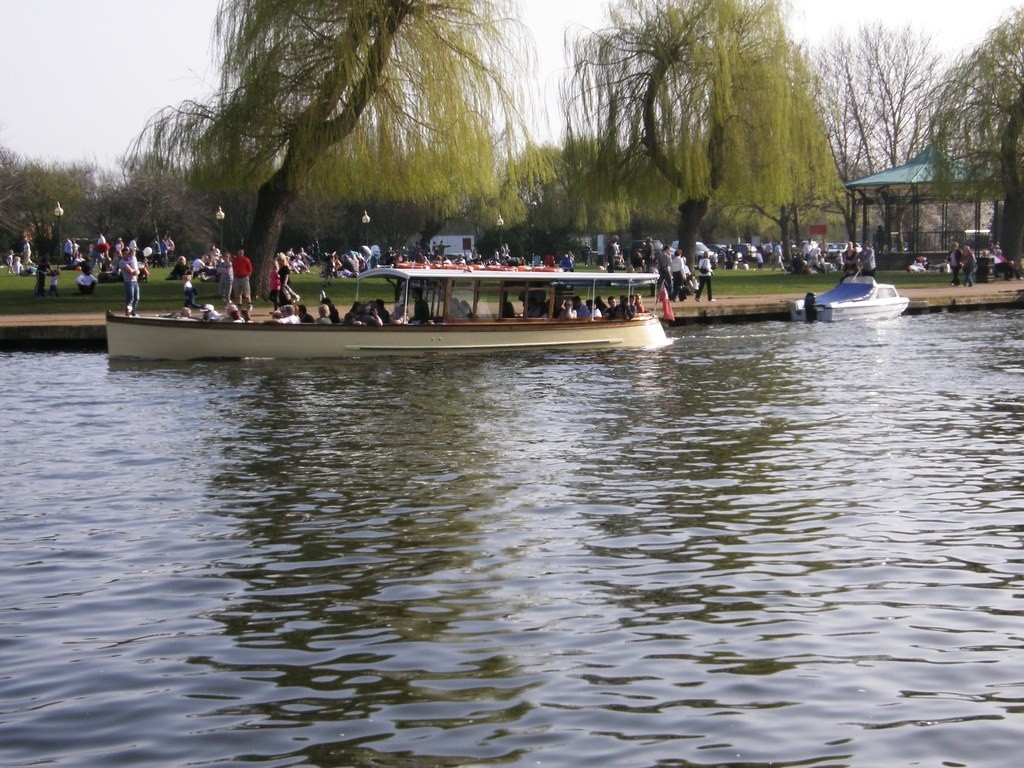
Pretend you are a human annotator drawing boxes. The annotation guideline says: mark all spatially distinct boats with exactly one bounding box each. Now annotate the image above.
[105,263,676,363]
[791,276,911,327]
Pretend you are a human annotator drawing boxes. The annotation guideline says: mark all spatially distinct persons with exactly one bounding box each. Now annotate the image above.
[502,292,652,321]
[606,234,715,302]
[269,247,310,314]
[560,250,575,272]
[321,240,526,292]
[178,298,409,326]
[582,241,590,266]
[411,287,429,323]
[982,242,1024,281]
[713,238,877,277]
[876,225,884,253]
[7,231,253,317]
[946,242,976,287]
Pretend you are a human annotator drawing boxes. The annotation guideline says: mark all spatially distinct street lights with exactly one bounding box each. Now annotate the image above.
[216,205,225,251]
[54,201,64,273]
[531,201,537,262]
[496,214,504,259]
[362,210,369,247]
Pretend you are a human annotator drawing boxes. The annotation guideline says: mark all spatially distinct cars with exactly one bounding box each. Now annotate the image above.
[672,240,719,270]
[708,245,743,264]
[623,239,677,267]
[827,242,850,258]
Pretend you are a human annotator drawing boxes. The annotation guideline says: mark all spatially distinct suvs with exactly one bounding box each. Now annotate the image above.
[733,243,757,258]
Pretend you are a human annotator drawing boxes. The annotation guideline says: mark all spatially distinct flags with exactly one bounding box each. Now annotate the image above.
[659,285,675,321]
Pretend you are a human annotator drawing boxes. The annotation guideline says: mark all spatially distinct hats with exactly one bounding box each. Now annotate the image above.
[396,302,404,308]
[205,304,214,312]
[319,305,330,316]
[285,304,294,316]
[228,305,238,315]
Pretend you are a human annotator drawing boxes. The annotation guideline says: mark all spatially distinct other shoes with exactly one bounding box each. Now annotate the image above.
[710,299,716,302]
[328,281,331,286]
[695,297,700,302]
[323,284,326,288]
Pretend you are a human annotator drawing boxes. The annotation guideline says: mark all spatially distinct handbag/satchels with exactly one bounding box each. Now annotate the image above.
[700,260,709,274]
